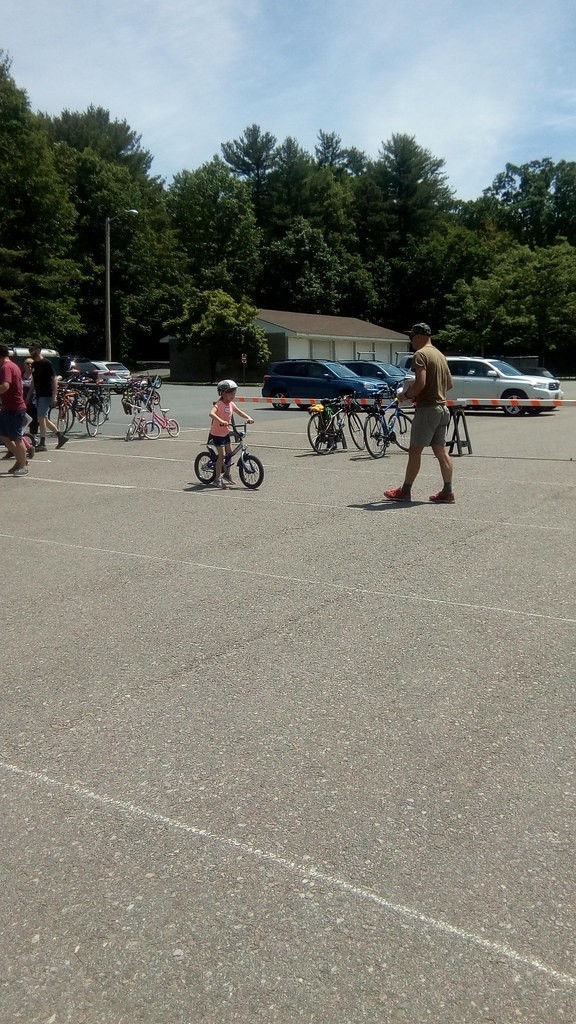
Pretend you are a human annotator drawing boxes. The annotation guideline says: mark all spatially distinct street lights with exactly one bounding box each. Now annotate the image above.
[106,210,138,362]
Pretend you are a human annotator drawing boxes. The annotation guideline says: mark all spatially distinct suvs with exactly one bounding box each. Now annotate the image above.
[335,360,406,390]
[61,355,98,382]
[262,358,389,410]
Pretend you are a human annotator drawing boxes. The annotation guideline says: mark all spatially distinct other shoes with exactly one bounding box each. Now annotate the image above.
[34,439,38,446]
[3,451,15,460]
[213,479,227,489]
[35,443,45,452]
[8,461,28,473]
[28,445,35,459]
[55,434,69,449]
[14,467,30,477]
[221,472,237,485]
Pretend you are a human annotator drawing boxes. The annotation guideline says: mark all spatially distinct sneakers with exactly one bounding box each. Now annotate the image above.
[429,491,456,503]
[384,486,411,502]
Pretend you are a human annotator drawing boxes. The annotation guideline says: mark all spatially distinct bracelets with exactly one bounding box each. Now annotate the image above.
[404,393,413,399]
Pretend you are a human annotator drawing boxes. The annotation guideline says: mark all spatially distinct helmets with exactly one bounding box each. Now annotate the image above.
[217,380,239,396]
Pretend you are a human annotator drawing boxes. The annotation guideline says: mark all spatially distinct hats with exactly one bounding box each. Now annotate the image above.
[28,343,41,350]
[404,323,432,337]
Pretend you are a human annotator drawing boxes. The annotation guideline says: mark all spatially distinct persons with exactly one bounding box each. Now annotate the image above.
[208,379,254,488]
[384,323,455,503]
[0,342,69,477]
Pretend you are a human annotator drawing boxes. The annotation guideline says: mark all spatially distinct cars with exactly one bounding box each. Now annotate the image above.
[91,361,133,394]
[8,347,63,381]
[396,353,563,416]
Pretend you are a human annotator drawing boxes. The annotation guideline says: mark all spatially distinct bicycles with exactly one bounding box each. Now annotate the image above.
[308,390,365,455]
[115,370,162,415]
[194,420,264,489]
[48,382,111,436]
[125,402,148,442]
[143,396,180,440]
[364,378,412,458]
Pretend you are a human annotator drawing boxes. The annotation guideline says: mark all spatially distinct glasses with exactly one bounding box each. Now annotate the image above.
[409,333,419,340]
[29,349,34,351]
